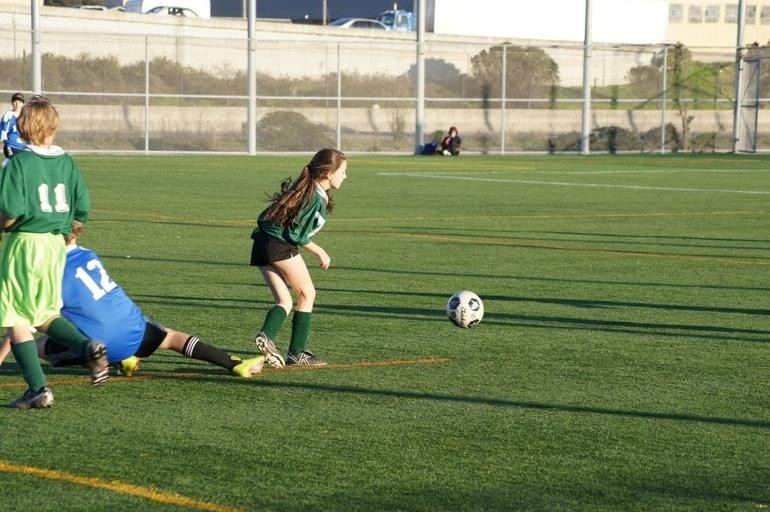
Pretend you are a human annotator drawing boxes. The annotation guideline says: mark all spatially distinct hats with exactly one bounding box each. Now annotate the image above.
[12,93,24,104]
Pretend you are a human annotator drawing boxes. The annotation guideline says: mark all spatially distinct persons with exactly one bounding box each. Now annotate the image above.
[0,93,29,160]
[441,127,462,156]
[0,95,109,410]
[0,231,266,378]
[249,149,347,369]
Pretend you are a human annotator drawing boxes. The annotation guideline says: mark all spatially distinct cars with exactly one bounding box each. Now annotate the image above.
[77,5,128,13]
[145,6,201,18]
[328,18,389,31]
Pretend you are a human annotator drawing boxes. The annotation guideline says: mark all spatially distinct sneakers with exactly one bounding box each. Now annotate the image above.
[285,350,328,366]
[84,339,111,389]
[230,355,266,379]
[118,355,141,377]
[254,332,286,369]
[9,385,54,410]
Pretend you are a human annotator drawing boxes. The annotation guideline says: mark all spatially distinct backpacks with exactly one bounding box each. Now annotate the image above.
[424,141,443,155]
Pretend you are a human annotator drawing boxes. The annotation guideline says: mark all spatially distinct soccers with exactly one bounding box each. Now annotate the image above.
[447,290,484,329]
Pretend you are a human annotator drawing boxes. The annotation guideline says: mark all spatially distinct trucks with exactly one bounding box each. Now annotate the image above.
[377,10,416,33]
